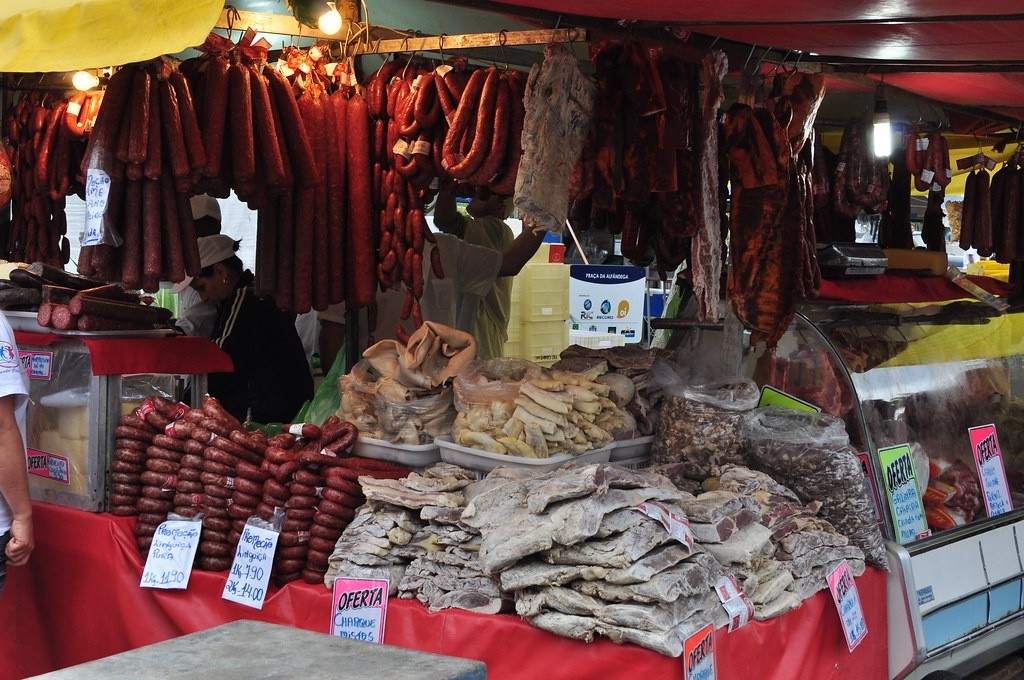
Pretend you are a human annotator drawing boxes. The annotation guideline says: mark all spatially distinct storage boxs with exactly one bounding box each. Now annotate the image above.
[503,262,626,369]
[643,288,670,317]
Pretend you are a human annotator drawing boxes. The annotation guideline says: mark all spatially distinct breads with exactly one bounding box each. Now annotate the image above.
[30,402,141,500]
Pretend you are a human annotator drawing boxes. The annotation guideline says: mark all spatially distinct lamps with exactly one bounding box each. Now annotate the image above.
[872,72,893,157]
[288,0,342,36]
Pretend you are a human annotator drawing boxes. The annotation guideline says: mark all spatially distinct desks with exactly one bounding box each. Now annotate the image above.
[0,500,889,680]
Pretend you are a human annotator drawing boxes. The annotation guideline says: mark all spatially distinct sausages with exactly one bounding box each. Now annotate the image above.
[0,45,651,345]
[108,397,424,585]
[797,116,1024,299]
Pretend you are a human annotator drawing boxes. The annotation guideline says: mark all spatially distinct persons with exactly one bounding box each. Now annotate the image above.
[175,194,249,425]
[170,234,315,425]
[0,311,35,593]
[433,186,514,360]
[318,224,548,377]
